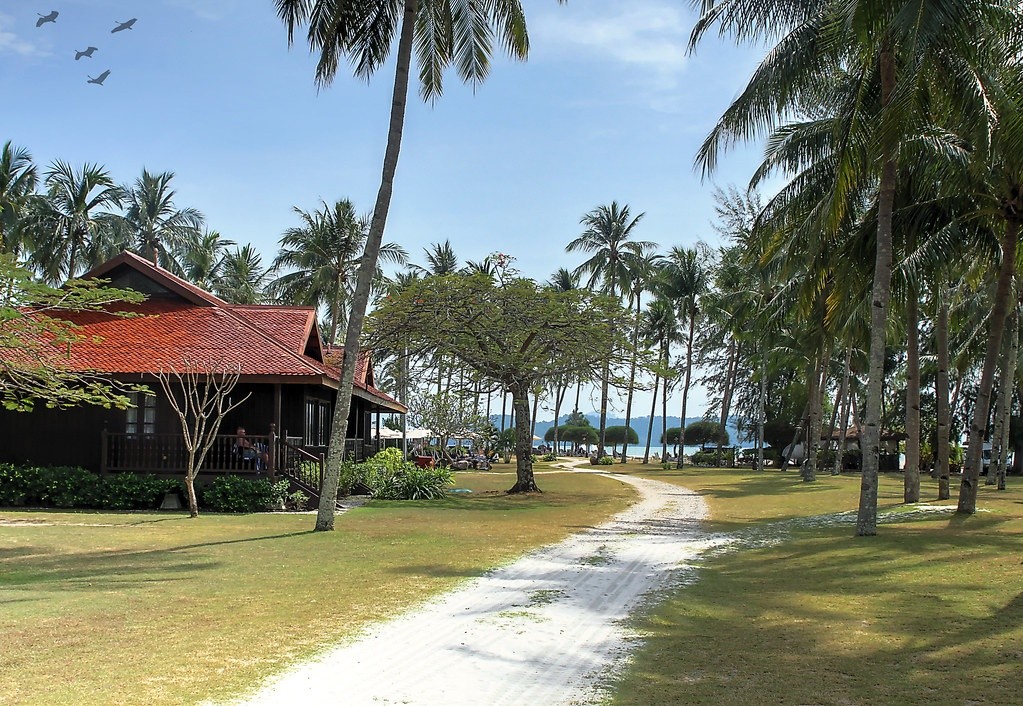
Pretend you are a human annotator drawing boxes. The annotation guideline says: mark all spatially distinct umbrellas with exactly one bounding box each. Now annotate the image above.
[371,426,482,459]
[533,435,542,446]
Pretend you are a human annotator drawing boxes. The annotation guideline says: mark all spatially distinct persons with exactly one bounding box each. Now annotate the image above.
[667,452,671,457]
[538,444,552,455]
[578,447,606,454]
[655,452,659,458]
[233,428,269,470]
[457,448,500,464]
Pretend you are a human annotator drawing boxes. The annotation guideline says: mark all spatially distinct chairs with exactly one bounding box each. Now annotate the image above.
[239,445,261,471]
